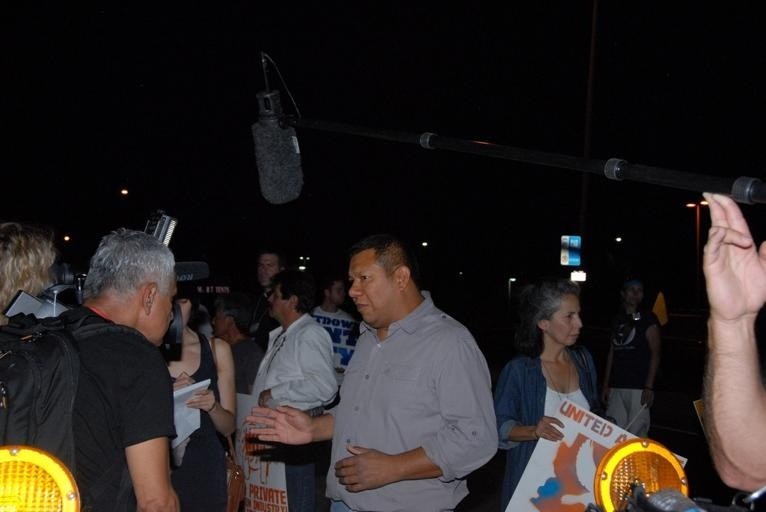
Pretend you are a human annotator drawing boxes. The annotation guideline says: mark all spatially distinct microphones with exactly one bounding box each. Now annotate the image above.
[250,50,305,205]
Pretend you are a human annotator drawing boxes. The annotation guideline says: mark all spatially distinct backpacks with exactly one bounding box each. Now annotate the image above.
[0,307,150,480]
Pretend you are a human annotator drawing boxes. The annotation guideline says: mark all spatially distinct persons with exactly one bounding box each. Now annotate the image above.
[2,222,260,512]
[491,272,659,511]
[248,236,499,510]
[703,192,764,511]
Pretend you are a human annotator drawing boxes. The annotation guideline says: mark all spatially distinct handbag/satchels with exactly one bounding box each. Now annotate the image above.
[224,455,246,512]
